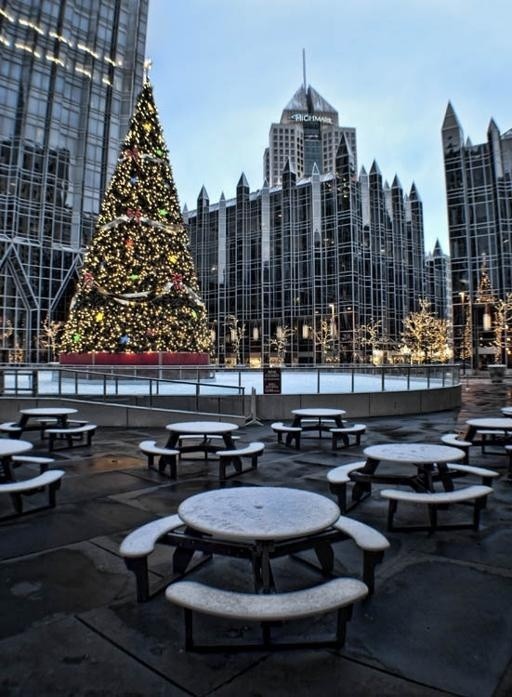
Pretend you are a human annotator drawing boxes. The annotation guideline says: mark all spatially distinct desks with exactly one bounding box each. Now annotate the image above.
[1,438,34,477]
[351,443,466,501]
[172,486,340,596]
[461,418,512,458]
[285,409,349,447]
[16,408,79,448]
[158,421,242,474]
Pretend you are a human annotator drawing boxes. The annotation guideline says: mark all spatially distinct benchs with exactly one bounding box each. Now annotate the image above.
[334,516,391,604]
[329,424,367,450]
[12,455,55,492]
[216,442,265,480]
[381,485,494,532]
[1,422,21,439]
[327,461,372,515]
[35,418,89,441]
[139,440,180,481]
[177,435,241,459]
[300,418,348,439]
[1,469,66,514]
[440,433,472,449]
[44,424,98,452]
[164,578,369,653]
[119,514,214,604]
[478,429,511,453]
[271,422,302,449]
[433,463,499,509]
